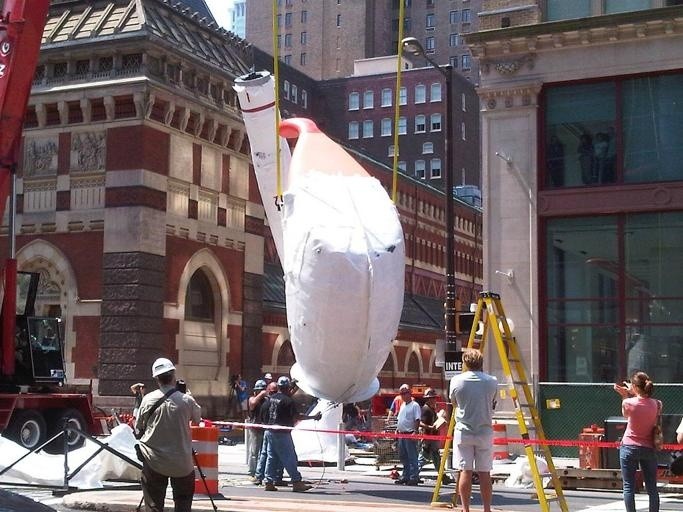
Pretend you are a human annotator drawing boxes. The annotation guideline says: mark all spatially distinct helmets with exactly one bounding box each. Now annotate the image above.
[424,388,439,398]
[278,376,291,387]
[152,358,176,377]
[270,382,278,391]
[265,372,272,379]
[253,380,267,390]
[399,383,411,394]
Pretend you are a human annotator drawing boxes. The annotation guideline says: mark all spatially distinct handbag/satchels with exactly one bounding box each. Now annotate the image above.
[671,455,683,476]
[654,399,665,454]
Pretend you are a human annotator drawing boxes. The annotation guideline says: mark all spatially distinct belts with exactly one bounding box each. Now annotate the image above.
[399,432,414,434]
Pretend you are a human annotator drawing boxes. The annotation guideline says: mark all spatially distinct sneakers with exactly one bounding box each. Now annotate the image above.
[443,478,452,484]
[277,481,287,485]
[408,480,416,484]
[256,481,263,485]
[395,480,408,483]
[293,482,312,491]
[266,484,277,491]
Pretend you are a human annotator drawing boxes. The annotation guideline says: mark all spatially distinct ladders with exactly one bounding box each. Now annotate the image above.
[431,292,569,512]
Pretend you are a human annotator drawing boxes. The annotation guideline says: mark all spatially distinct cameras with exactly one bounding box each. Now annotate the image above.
[138,384,144,389]
[176,379,187,394]
[618,378,631,388]
[229,374,236,385]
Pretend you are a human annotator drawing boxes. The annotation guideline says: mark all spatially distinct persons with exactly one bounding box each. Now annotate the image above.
[415,388,455,485]
[262,376,322,492]
[614,371,663,512]
[253,381,279,486]
[247,380,267,477]
[234,373,248,420]
[395,386,421,486]
[449,349,498,512]
[389,384,415,415]
[134,358,201,512]
[676,417,683,445]
[130,383,147,419]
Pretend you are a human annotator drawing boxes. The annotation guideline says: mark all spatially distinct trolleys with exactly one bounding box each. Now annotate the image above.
[371,414,399,470]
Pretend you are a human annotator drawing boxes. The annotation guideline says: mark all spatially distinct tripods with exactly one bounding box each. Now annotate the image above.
[226,385,243,420]
[136,447,218,512]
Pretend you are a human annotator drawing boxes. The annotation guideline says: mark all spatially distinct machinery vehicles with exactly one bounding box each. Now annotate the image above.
[1,3,95,454]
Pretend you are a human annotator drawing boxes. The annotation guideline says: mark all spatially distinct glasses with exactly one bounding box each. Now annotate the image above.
[400,392,409,395]
